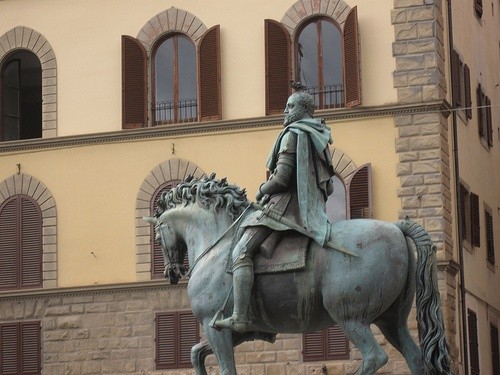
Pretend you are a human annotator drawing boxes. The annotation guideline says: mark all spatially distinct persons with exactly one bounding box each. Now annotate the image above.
[212,89,336,333]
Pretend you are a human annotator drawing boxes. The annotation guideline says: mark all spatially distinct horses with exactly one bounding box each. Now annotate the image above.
[143,172,452,374]
